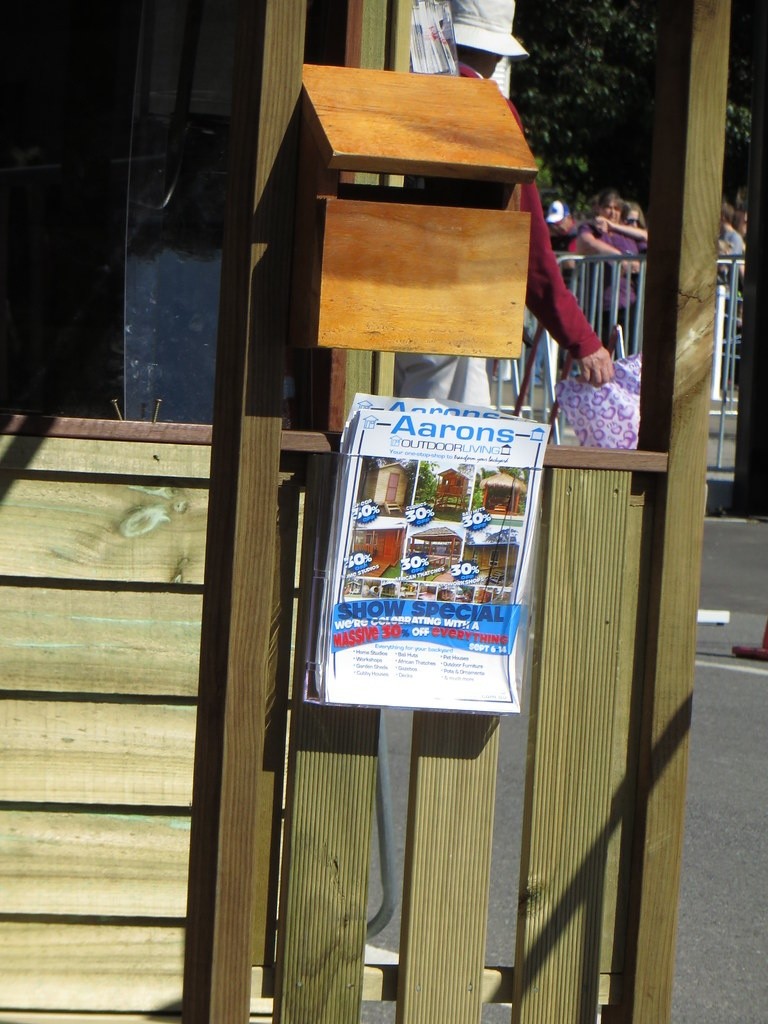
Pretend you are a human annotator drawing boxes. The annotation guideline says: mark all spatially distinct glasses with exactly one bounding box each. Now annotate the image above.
[601,204,621,211]
[624,218,647,229]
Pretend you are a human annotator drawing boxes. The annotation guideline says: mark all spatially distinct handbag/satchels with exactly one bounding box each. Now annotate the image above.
[555,352,642,450]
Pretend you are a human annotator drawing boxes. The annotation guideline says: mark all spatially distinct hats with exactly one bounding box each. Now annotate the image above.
[450,0,530,62]
[545,200,570,223]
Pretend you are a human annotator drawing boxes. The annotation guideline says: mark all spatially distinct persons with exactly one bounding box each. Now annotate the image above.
[531,188,749,387]
[395,0,615,406]
